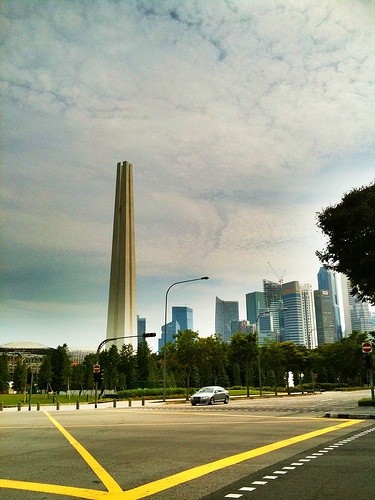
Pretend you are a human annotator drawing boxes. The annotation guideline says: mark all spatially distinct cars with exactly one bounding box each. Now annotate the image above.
[190,386,230,406]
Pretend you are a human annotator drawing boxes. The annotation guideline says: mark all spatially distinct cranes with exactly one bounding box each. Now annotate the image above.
[267,261,285,343]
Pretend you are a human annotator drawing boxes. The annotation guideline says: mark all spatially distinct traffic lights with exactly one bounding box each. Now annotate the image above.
[27,373,31,384]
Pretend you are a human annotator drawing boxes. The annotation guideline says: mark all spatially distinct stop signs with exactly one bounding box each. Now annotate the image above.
[93,365,100,372]
[362,343,372,353]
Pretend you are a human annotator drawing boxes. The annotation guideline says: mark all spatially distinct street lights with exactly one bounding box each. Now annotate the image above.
[256,307,289,396]
[163,276,209,402]
